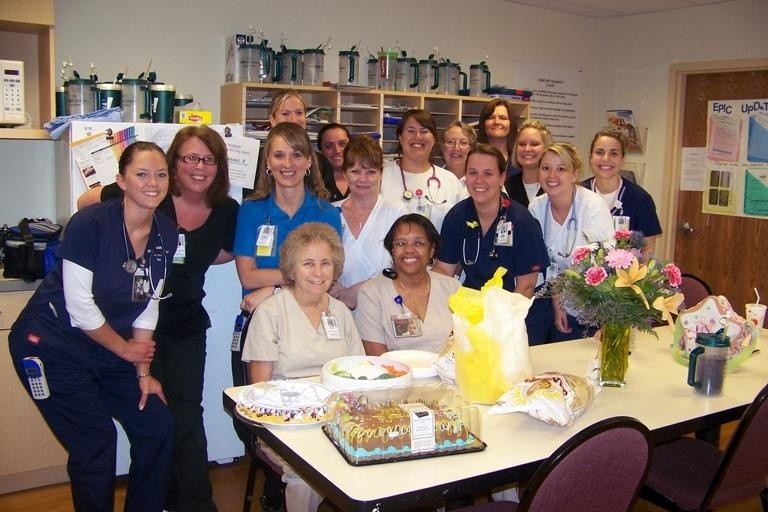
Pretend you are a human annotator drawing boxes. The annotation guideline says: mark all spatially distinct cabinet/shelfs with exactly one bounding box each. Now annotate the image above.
[1,279,75,497]
[217,81,533,179]
[1,0,60,144]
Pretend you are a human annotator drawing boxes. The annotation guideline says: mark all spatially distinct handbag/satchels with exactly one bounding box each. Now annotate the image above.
[1,216,65,285]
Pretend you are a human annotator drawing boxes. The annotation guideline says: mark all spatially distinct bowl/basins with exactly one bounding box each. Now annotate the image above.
[321,357,413,406]
[382,351,445,379]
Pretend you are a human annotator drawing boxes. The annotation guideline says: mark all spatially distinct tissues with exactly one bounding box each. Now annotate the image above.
[178,102,213,126]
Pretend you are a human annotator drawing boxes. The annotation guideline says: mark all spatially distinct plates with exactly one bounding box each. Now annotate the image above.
[333,84,374,90]
[236,399,333,429]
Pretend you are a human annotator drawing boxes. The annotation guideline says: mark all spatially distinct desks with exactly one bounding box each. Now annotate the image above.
[220,311,768,512]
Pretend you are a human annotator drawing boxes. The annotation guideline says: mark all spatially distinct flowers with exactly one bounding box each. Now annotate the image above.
[529,223,684,381]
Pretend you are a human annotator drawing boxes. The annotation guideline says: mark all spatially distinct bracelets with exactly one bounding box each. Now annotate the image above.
[135,372,151,378]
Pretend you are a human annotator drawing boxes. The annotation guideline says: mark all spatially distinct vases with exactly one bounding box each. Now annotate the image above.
[593,316,632,388]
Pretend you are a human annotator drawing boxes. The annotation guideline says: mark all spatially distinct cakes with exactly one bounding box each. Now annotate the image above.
[324,392,475,463]
[240,377,332,426]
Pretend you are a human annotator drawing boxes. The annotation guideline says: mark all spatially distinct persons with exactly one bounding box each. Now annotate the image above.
[76,124,241,512]
[8,141,179,512]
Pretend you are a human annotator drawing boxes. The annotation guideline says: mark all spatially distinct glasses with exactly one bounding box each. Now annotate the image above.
[390,239,432,248]
[176,156,219,166]
[442,138,472,149]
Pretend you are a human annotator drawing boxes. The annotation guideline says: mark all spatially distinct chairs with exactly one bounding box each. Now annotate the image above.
[651,272,714,325]
[634,383,767,512]
[445,410,654,512]
[237,305,302,510]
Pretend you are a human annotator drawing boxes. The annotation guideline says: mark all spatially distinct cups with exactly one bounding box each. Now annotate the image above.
[338,45,362,84]
[58,71,193,124]
[468,60,493,98]
[242,39,325,85]
[367,47,467,94]
[744,303,767,348]
[687,331,732,396]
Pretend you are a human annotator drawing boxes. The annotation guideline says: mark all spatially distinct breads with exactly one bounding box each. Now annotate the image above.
[489,370,592,426]
